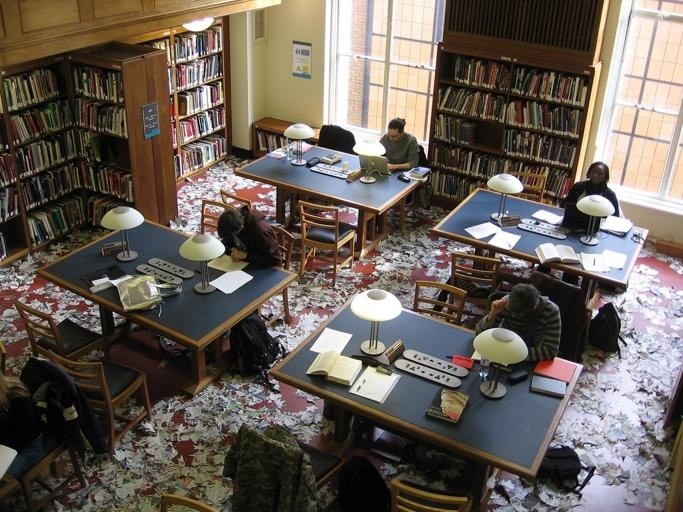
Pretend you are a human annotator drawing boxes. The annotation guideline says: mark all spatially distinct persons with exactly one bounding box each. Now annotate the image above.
[566,162,619,218]
[218,205,282,266]
[476,284,561,362]
[379,118,419,170]
[0,344,44,480]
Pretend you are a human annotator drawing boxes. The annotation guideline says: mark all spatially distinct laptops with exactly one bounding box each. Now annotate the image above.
[561,201,602,232]
[358,154,393,175]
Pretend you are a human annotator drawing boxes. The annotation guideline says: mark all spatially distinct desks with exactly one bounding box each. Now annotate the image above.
[35,219,298,397]
[268,295,584,512]
[234,141,429,262]
[431,187,650,308]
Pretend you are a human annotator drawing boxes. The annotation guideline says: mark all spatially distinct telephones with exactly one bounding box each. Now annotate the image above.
[320,153,343,164]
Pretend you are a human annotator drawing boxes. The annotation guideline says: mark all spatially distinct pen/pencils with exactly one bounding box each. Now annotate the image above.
[194,270,210,275]
[447,356,453,359]
[356,380,366,392]
[496,364,513,372]
[593,258,596,266]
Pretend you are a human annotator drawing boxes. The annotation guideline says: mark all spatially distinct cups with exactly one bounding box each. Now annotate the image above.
[479,359,489,378]
[633,226,643,240]
[282,139,293,160]
[342,160,349,172]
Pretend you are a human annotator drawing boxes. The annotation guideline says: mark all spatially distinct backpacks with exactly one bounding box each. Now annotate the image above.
[587,301,626,361]
[228,311,288,394]
[413,182,433,210]
[536,445,596,499]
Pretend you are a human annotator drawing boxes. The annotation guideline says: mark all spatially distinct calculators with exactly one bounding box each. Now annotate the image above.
[82,265,127,287]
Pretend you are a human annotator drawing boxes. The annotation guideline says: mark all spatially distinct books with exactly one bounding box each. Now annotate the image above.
[257,130,318,153]
[531,375,566,398]
[306,353,363,387]
[0,65,135,262]
[429,56,587,209]
[535,243,581,265]
[320,153,342,165]
[427,387,469,424]
[410,166,431,179]
[534,359,577,385]
[142,26,228,180]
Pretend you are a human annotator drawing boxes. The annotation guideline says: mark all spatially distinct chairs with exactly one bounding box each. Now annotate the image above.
[14,300,105,362]
[297,200,357,287]
[255,224,294,330]
[237,420,347,511]
[0,343,96,511]
[160,493,221,511]
[389,442,476,512]
[319,125,356,155]
[200,188,286,259]
[508,172,547,204]
[381,144,428,208]
[413,252,594,365]
[45,348,152,457]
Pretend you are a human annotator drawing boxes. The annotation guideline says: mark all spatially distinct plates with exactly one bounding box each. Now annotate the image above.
[359,176,376,184]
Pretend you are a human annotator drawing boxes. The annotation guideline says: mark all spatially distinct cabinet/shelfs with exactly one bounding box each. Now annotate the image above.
[140,14,232,183]
[425,0,610,212]
[251,117,321,157]
[0,44,179,266]
[0,0,284,65]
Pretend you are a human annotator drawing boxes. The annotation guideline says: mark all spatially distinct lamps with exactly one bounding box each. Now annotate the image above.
[576,195,615,247]
[180,234,226,294]
[100,206,144,261]
[472,328,528,399]
[351,288,403,356]
[283,123,315,166]
[352,140,387,184]
[487,174,523,220]
